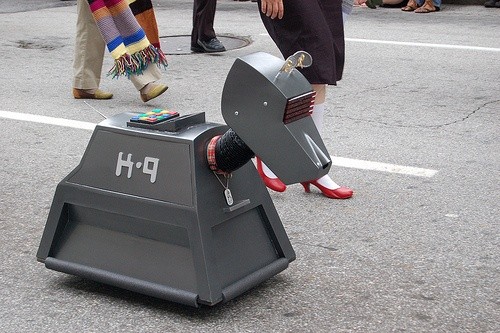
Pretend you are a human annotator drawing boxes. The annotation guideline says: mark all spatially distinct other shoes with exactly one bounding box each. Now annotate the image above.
[197,37,226,51]
[73,88,113,98]
[190,45,206,52]
[140,83,168,102]
[401,0,418,10]
[414,0,435,12]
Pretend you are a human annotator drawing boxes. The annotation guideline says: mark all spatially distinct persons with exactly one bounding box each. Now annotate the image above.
[189,0,226,54]
[399,0,442,13]
[71,0,170,103]
[253,0,368,200]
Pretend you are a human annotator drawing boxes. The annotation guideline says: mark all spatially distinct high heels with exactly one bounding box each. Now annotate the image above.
[257,156,286,191]
[299,176,354,198]
